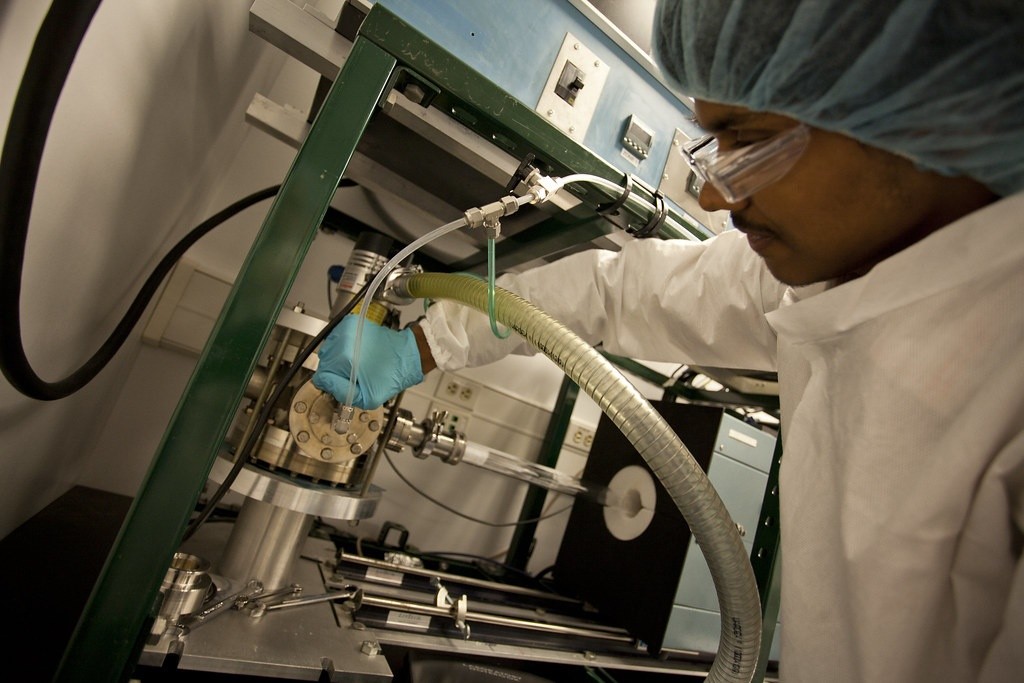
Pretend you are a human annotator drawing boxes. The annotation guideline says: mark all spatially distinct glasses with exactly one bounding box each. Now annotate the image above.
[679,124,812,205]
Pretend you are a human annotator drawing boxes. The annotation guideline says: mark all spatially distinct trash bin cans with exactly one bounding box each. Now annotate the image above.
[401,649,555,683]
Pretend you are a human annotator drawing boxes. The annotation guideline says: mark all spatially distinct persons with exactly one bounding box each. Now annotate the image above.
[310,1,1024,683]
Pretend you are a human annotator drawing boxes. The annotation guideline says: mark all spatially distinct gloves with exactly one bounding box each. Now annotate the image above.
[310,314,425,411]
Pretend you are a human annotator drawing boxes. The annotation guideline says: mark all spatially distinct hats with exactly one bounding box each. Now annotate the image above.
[648,1,1024,201]
